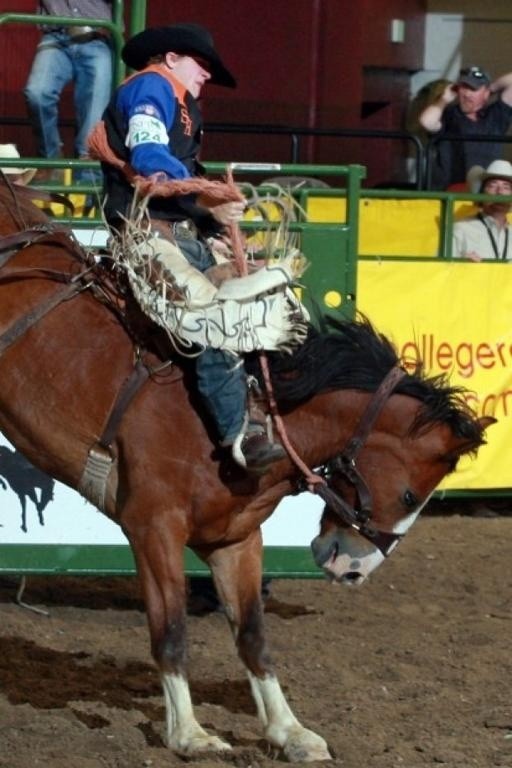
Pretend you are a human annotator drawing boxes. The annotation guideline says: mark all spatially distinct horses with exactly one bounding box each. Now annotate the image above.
[0,166,499,764]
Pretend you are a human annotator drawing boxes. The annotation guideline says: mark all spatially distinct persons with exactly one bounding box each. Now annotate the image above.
[100,20,286,481]
[419,67,512,191]
[450,159,512,263]
[23,0,113,183]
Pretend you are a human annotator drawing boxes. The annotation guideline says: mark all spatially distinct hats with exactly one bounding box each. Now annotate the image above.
[122,23,236,88]
[467,159,512,193]
[457,66,488,89]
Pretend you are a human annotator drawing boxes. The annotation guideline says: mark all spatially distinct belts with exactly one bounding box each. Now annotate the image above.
[68,26,115,44]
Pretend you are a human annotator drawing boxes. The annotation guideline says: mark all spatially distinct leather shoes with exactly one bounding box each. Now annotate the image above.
[231,435,285,476]
